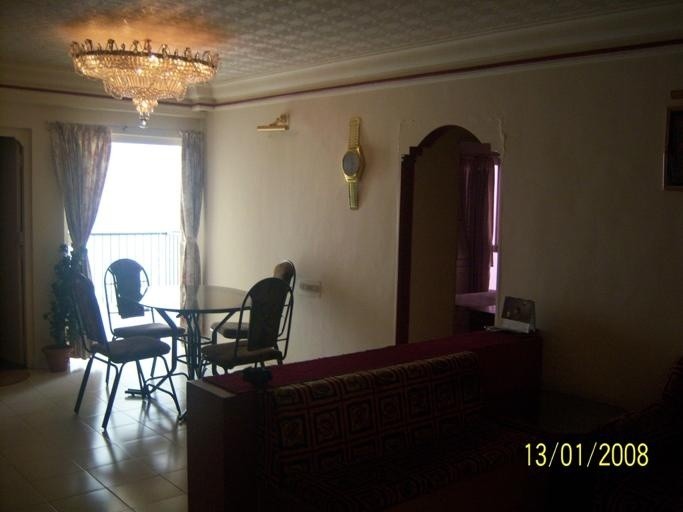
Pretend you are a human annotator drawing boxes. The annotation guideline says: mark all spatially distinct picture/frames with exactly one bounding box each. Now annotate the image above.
[659,105,683,191]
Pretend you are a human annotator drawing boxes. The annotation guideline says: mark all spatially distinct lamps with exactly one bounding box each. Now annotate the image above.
[70,38,220,129]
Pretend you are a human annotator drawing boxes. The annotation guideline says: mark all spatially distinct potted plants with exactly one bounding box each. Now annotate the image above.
[41,245,86,371]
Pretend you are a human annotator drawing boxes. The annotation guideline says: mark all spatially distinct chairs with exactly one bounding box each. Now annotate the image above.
[69,258,296,429]
